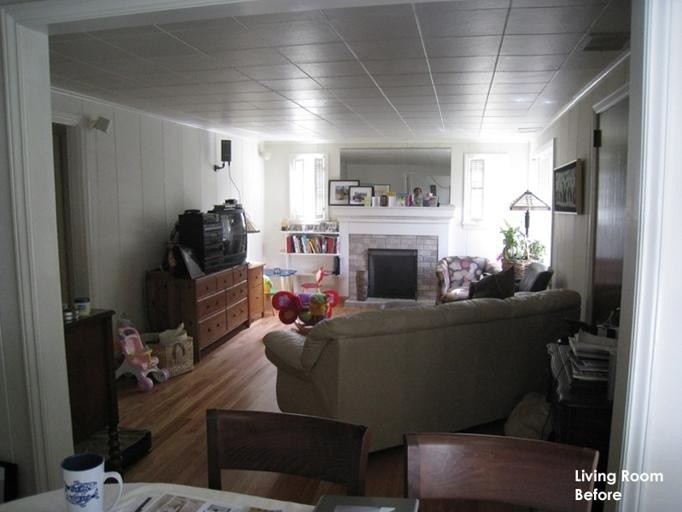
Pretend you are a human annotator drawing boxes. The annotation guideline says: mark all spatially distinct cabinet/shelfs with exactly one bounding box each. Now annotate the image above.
[247,263,265,326]
[147,263,249,363]
[279,231,341,298]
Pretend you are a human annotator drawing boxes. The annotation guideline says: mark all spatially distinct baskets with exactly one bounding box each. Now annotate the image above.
[148,337,196,378]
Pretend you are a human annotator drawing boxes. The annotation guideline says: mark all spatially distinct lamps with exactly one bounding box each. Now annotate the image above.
[213,140,231,171]
[88,116,110,133]
[246,215,259,232]
[511,191,550,238]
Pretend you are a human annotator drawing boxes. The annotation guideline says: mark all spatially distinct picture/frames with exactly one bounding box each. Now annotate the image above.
[328,180,391,207]
[552,159,583,215]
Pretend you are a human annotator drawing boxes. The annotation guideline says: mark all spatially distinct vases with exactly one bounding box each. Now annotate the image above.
[356,270,369,300]
[292,319,314,335]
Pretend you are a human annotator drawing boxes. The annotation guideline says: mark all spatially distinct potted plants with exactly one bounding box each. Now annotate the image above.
[497,217,545,286]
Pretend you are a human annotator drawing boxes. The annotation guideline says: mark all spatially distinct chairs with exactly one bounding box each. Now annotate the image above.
[467,265,514,299]
[402,433,600,511]
[517,262,554,292]
[204,407,366,497]
[436,256,502,304]
[300,266,324,294]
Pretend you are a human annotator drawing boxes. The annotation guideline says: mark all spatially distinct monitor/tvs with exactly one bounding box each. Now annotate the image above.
[215,211,249,268]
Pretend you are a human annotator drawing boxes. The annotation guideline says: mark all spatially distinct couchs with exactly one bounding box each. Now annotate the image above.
[263,288,581,454]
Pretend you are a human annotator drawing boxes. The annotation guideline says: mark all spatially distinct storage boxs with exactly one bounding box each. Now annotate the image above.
[139,332,196,378]
[263,268,297,294]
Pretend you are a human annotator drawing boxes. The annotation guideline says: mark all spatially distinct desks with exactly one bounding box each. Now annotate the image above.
[544,342,613,444]
[64,308,119,449]
[0,482,329,512]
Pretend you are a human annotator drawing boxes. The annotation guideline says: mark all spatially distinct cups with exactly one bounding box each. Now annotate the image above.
[61,452,123,512]
[370,191,439,208]
[74,297,90,317]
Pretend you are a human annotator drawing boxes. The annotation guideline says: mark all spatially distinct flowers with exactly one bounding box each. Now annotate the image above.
[271,290,340,323]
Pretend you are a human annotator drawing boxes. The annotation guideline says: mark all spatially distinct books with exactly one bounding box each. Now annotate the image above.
[286,235,339,253]
[566,328,617,381]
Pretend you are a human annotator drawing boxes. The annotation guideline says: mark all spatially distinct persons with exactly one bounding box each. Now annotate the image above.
[273,290,338,326]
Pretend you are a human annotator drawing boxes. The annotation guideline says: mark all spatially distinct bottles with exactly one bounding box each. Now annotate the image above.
[63,309,80,322]
